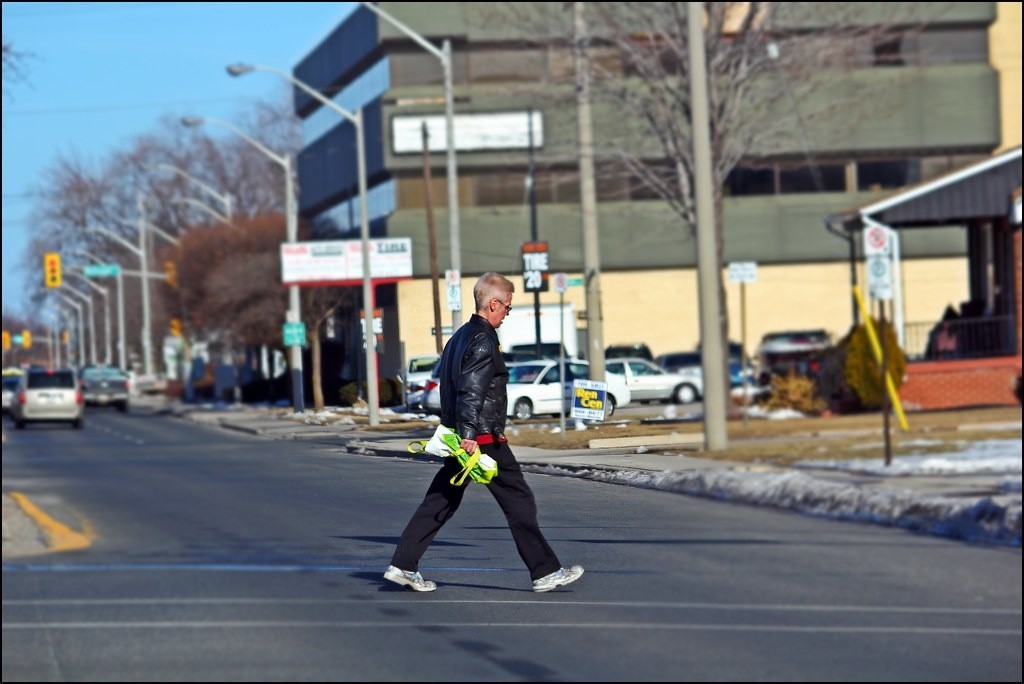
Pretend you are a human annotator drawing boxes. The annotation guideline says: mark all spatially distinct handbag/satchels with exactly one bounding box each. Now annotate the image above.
[407,423,498,486]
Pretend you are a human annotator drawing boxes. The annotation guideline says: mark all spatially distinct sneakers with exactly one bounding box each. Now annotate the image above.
[532,565,585,592]
[383,564,437,592]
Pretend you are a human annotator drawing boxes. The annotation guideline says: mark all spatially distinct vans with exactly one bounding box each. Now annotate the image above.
[402,352,441,411]
[14,365,84,426]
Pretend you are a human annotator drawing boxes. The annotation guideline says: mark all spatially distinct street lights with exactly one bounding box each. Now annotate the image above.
[46,216,153,397]
[155,163,244,404]
[228,63,383,428]
[183,117,304,414]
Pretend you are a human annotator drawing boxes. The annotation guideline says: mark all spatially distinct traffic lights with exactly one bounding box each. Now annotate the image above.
[46,253,60,287]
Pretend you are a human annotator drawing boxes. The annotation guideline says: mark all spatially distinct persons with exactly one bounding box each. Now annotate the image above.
[384,273,585,592]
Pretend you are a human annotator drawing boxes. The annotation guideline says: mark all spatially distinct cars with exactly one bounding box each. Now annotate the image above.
[702,344,758,391]
[505,358,630,419]
[761,330,833,383]
[605,345,707,406]
[426,356,443,416]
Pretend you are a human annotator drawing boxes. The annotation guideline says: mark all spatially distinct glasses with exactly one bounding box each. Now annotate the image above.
[496,298,513,312]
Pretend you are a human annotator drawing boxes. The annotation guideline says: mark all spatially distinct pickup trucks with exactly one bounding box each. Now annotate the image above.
[80,364,131,410]
